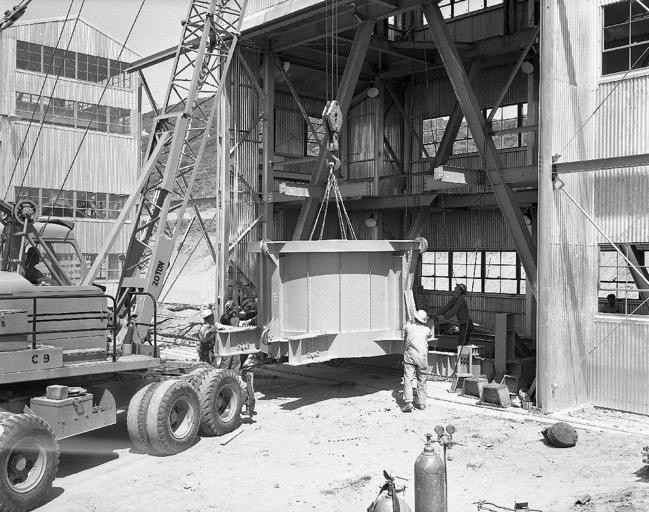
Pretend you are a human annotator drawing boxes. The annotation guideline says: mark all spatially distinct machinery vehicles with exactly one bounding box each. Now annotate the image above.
[0,198,249,512]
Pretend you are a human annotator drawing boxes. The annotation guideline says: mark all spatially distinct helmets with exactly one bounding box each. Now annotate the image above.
[456,283,467,295]
[414,309,427,324]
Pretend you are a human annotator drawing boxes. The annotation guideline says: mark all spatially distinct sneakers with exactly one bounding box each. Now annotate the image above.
[401,402,414,412]
[417,404,425,410]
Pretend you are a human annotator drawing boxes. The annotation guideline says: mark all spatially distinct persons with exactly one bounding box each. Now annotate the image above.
[435,283,474,344]
[401,309,433,412]
[600,294,621,313]
[220,296,257,381]
[197,309,225,366]
[24,247,51,286]
